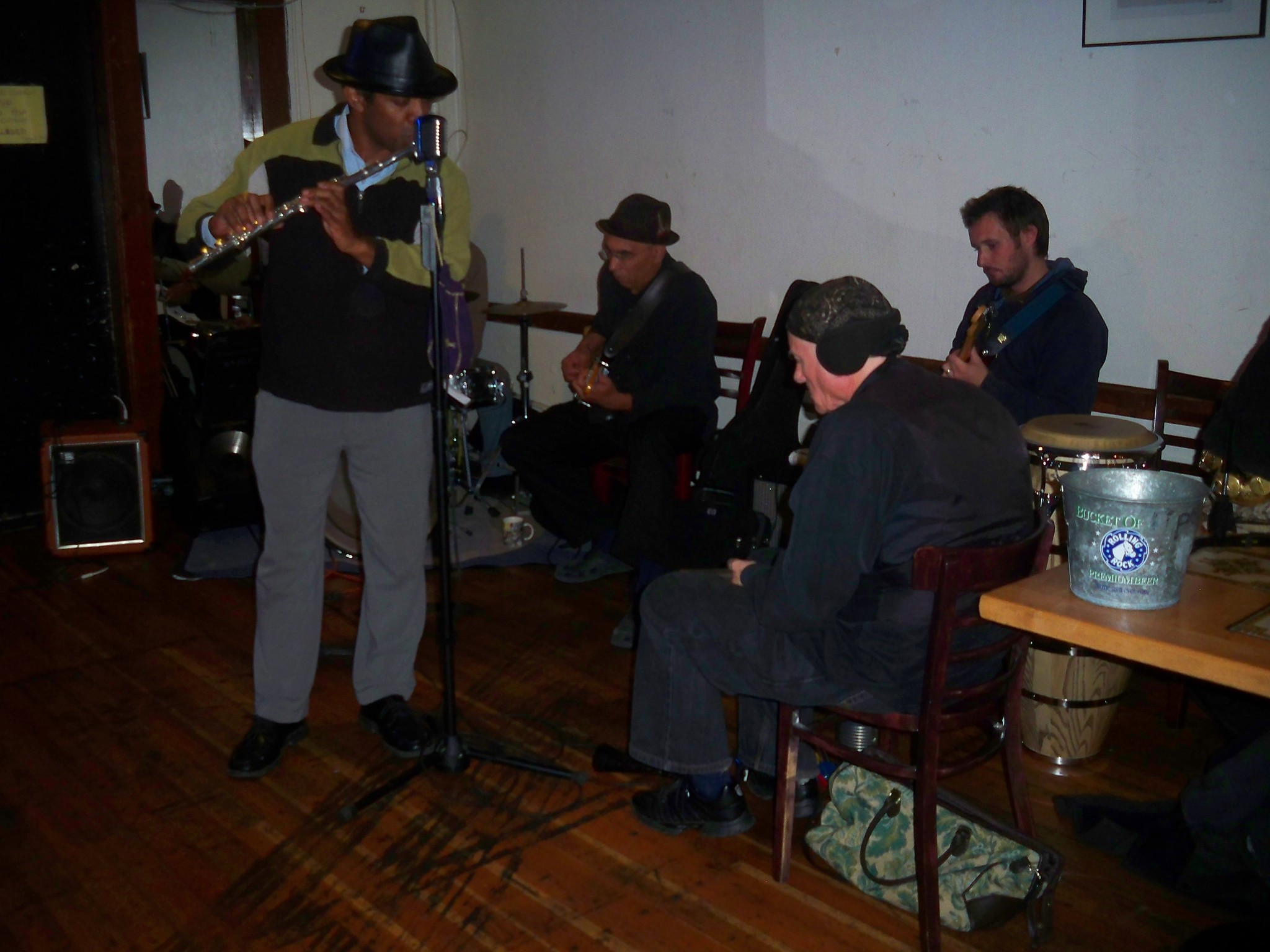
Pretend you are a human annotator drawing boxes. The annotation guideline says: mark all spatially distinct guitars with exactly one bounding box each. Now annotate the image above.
[948,307,996,379]
[572,350,625,409]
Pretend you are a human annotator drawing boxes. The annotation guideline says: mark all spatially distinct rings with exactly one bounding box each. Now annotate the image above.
[946,369,951,374]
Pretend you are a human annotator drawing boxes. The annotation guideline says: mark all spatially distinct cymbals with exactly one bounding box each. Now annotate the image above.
[486,299,568,318]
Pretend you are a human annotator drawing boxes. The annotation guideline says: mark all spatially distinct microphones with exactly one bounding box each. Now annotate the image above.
[411,114,447,221]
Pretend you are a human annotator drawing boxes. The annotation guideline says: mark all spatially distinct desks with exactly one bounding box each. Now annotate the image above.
[979,528,1270,702]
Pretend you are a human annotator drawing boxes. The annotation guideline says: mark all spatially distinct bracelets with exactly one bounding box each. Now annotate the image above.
[789,449,799,466]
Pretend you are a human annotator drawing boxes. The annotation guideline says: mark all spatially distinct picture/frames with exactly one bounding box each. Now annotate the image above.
[1081,0,1266,48]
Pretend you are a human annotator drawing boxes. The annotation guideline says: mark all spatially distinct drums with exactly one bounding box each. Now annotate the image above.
[320,451,443,553]
[1017,413,1166,764]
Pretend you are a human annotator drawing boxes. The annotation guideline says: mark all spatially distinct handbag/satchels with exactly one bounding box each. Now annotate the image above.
[803,761,1062,948]
[677,277,822,568]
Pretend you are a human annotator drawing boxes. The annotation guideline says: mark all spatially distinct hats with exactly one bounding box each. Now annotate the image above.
[786,275,906,355]
[596,193,679,245]
[323,16,458,99]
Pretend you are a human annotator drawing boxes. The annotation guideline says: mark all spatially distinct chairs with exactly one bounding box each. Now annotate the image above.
[591,316,769,502]
[1150,359,1236,480]
[776,518,1056,952]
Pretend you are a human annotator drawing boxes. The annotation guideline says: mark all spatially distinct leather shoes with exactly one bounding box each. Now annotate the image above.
[359,694,435,758]
[227,716,308,777]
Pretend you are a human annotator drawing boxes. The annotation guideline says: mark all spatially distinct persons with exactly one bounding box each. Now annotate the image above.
[149,190,259,428]
[626,277,1038,839]
[439,194,820,648]
[1053,315,1269,899]
[943,185,1109,427]
[181,16,485,779]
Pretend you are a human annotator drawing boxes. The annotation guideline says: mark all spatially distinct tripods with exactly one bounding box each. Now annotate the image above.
[341,211,588,824]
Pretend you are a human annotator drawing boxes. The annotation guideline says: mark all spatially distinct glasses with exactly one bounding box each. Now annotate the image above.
[597,246,654,263]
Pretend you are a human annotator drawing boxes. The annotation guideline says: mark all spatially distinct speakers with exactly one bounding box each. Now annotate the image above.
[40,431,153,556]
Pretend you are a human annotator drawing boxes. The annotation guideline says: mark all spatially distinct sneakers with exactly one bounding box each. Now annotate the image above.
[739,762,817,820]
[557,548,635,582]
[610,605,639,648]
[632,774,754,838]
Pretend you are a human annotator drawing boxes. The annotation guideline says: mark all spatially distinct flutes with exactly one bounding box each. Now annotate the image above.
[183,144,414,277]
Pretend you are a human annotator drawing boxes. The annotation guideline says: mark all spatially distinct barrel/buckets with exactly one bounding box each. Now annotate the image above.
[1018,638,1136,767]
[1056,467,1210,611]
